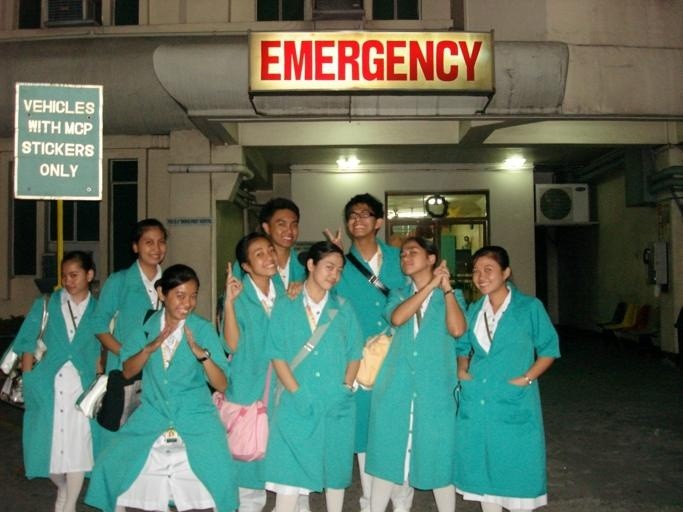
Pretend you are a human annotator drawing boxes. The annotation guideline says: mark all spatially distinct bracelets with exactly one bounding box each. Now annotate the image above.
[443,289,454,295]
[523,375,533,385]
[342,382,353,389]
[196,348,210,362]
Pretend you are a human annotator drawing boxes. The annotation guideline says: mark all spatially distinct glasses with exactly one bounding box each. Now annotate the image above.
[347,210,380,219]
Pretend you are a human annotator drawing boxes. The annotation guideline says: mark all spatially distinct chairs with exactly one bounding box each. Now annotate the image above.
[595,299,661,352]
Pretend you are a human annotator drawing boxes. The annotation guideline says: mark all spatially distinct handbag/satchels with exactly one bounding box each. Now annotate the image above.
[74,373,109,420]
[96,369,143,432]
[355,325,395,388]
[0,295,49,411]
[211,391,269,462]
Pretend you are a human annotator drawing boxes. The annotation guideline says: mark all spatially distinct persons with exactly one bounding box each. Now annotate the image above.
[321,191,413,512]
[94,263,241,512]
[452,244,562,510]
[12,251,106,510]
[230,197,308,291]
[262,240,363,512]
[215,232,284,512]
[93,217,169,366]
[363,234,466,509]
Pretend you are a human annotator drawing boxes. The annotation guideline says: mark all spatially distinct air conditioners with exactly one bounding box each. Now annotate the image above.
[535,183,589,225]
